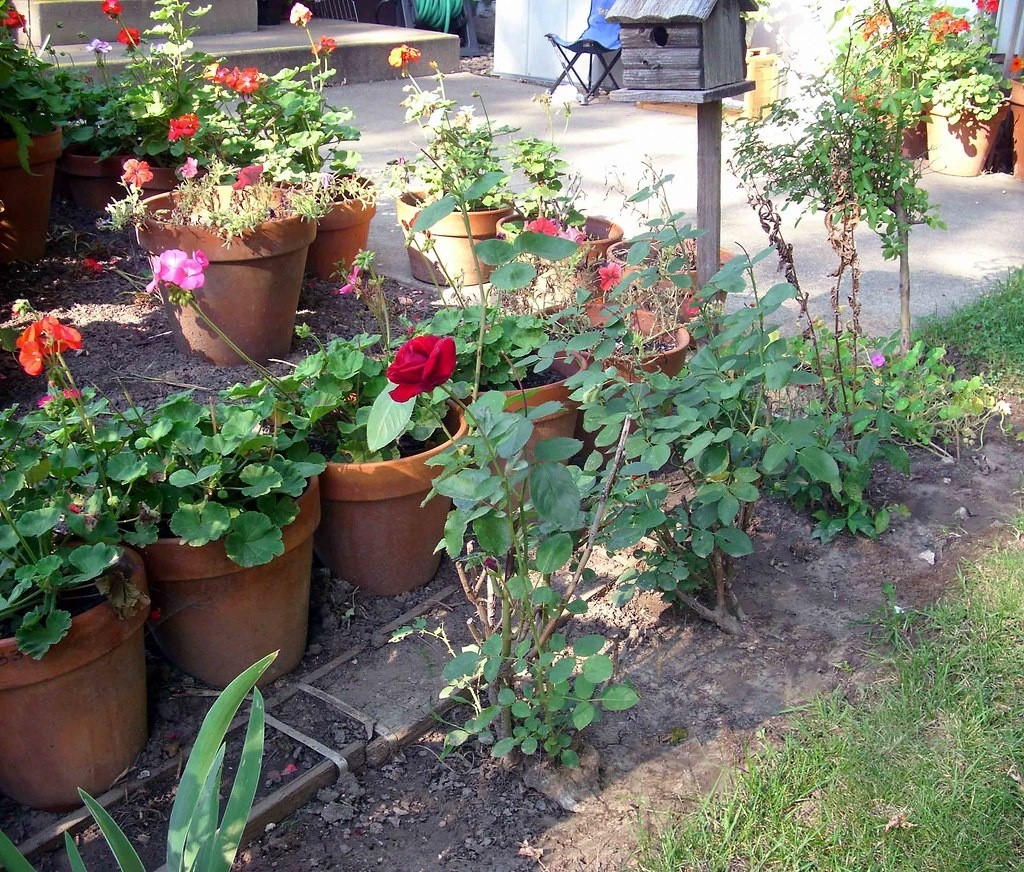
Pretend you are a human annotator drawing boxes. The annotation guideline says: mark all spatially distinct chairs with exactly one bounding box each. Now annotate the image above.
[544,0,622,106]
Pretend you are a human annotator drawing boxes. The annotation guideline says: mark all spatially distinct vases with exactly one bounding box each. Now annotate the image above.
[464,353,589,519]
[926,100,1009,177]
[874,100,927,158]
[395,193,514,286]
[1009,79,1024,179]
[0,127,380,364]
[316,397,474,595]
[125,463,321,690]
[0,547,149,814]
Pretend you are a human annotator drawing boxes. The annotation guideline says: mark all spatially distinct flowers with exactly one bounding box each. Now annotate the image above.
[829,0,1024,125]
[1,0,384,244]
[0,215,552,661]
[391,46,509,211]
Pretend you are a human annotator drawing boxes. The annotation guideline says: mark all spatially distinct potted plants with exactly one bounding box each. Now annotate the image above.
[494,245,689,472]
[496,87,624,309]
[607,159,735,350]
